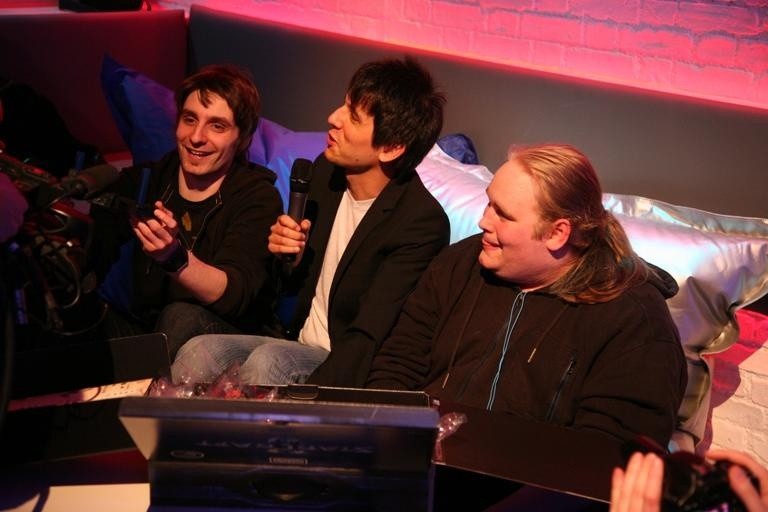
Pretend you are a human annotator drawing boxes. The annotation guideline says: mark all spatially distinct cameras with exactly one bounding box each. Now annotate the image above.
[621,433,762,511]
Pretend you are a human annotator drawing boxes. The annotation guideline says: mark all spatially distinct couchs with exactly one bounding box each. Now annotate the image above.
[185,1,767,466]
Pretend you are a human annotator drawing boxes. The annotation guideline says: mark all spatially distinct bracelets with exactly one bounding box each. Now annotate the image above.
[161,238,189,274]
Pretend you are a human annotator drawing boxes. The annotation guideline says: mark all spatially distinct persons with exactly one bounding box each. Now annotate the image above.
[609,447,768,511]
[169,54,452,388]
[363,140,688,502]
[51,60,285,378]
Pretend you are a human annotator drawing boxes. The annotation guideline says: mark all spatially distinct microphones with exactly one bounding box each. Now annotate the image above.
[282,158,314,261]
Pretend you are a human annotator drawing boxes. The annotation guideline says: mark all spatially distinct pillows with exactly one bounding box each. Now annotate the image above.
[411,139,768,428]
[96,42,479,218]
